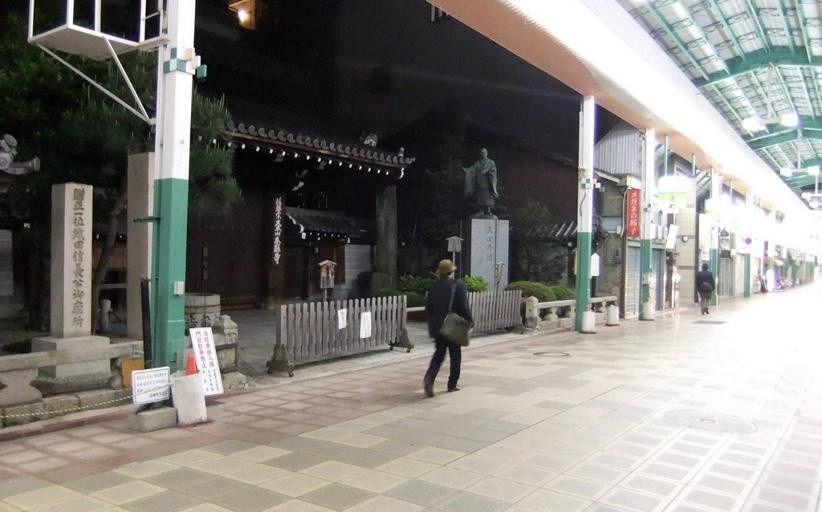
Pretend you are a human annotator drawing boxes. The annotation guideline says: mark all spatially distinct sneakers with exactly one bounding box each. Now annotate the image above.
[423,373,435,398]
[447,386,461,392]
[701,308,710,316]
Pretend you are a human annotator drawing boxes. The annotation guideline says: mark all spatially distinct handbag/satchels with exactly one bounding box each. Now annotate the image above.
[437,312,474,348]
[699,281,713,293]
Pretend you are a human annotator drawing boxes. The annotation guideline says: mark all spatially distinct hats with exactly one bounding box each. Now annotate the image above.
[435,258,457,275]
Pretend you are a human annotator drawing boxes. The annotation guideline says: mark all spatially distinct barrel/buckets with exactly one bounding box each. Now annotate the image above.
[582,309,596,333]
[642,302,653,319]
[170,368,210,428]
[607,306,619,326]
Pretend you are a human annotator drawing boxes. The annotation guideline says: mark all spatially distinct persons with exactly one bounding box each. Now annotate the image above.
[459,145,502,217]
[694,263,716,316]
[419,258,476,399]
